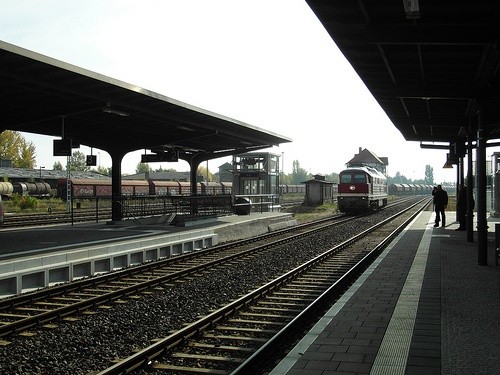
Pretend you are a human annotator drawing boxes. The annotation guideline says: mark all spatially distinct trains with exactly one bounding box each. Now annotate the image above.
[337,164,388,212]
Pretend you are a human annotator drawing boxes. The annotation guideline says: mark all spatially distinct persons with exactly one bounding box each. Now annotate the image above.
[454,184,474,231]
[432,185,448,228]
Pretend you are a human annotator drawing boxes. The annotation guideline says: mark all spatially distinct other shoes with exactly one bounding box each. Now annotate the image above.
[455,228,464,230]
[434,224,439,227]
[442,223,445,226]
